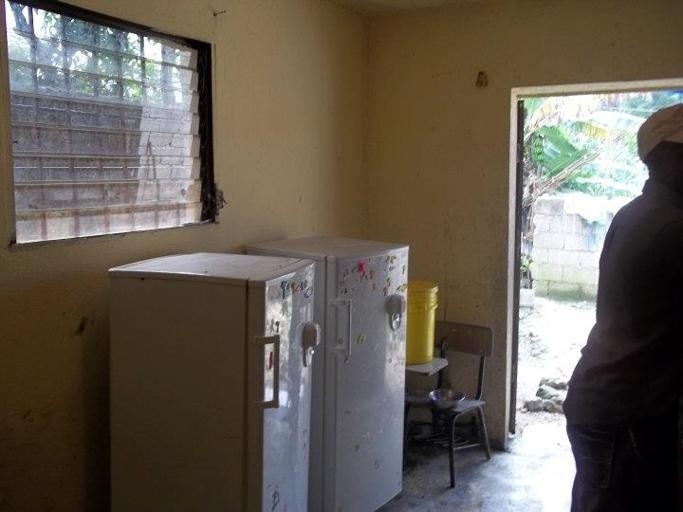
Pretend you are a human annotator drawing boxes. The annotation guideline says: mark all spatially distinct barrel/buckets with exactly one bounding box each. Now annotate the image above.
[406,279,440,367]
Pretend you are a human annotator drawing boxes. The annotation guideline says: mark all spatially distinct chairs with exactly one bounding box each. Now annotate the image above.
[402,319,493,489]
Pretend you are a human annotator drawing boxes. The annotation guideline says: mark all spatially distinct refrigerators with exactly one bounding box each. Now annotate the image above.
[238,234,412,512]
[106,252,321,512]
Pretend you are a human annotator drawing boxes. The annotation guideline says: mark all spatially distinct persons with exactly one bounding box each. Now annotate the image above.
[562,101,683,512]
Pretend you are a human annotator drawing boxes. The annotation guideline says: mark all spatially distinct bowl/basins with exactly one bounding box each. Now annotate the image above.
[428,388,467,410]
